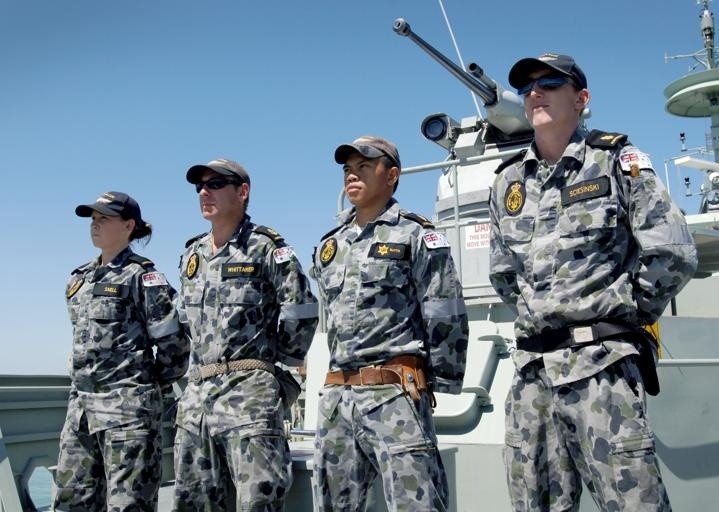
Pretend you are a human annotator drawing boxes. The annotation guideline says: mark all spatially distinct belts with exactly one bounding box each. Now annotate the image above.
[324,363,403,390]
[520,319,663,395]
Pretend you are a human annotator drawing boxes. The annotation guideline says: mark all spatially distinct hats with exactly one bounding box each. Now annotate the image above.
[76,191,141,221]
[334,134,401,172]
[186,159,250,193]
[508,53,587,89]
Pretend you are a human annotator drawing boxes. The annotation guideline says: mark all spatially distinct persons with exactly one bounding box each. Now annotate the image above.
[49,190,188,512]
[310,135,470,511]
[487,51,701,511]
[170,158,321,512]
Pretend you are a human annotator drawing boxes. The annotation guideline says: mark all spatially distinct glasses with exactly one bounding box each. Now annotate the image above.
[518,74,580,95]
[196,177,240,192]
